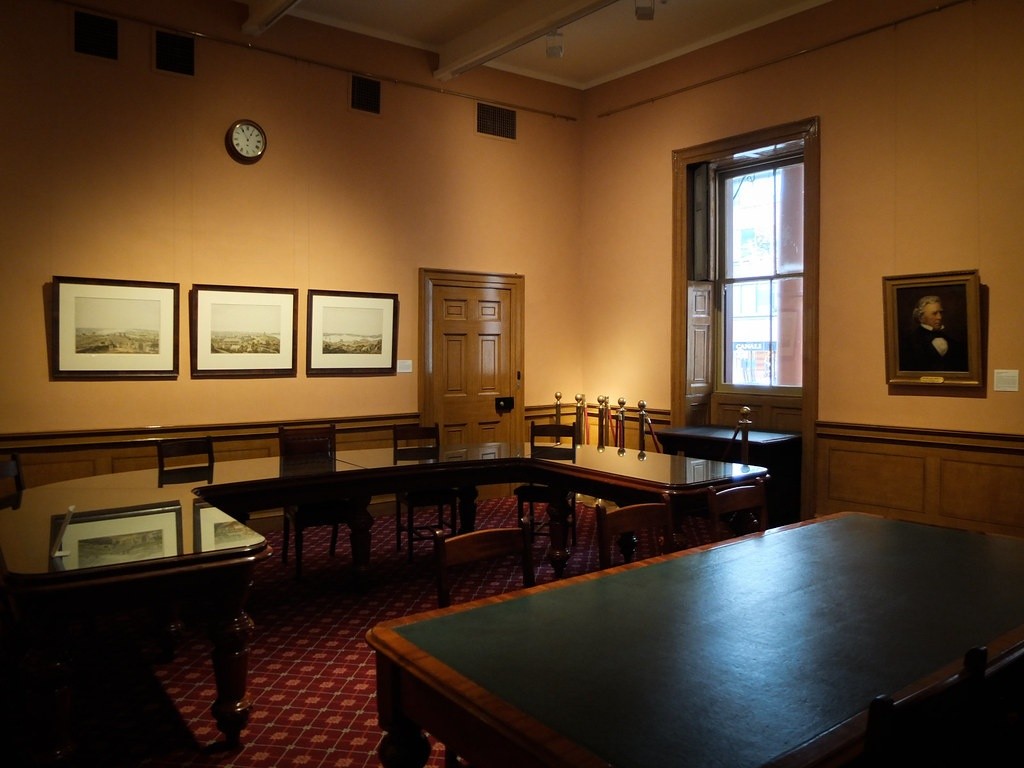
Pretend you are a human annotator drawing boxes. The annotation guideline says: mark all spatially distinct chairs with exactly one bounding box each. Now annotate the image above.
[707,475,767,541]
[391,421,462,554]
[0,491,23,510]
[0,453,30,491]
[158,462,214,488]
[279,423,361,579]
[596,491,682,567]
[511,421,578,548]
[862,644,988,768]
[154,436,252,527]
[532,443,576,464]
[431,515,535,608]
[395,448,440,464]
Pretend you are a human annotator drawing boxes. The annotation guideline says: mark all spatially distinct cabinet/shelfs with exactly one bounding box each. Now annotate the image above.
[644,424,802,529]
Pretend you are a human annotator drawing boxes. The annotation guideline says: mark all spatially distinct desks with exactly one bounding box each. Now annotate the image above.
[365,507,1024,768]
[0,439,771,768]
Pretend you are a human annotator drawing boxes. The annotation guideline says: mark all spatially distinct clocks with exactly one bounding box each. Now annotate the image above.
[227,118,267,162]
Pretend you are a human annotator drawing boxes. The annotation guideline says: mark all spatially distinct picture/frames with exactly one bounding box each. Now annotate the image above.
[191,284,298,375]
[192,498,265,552]
[50,499,183,573]
[51,275,179,378]
[306,288,399,376]
[881,268,987,390]
